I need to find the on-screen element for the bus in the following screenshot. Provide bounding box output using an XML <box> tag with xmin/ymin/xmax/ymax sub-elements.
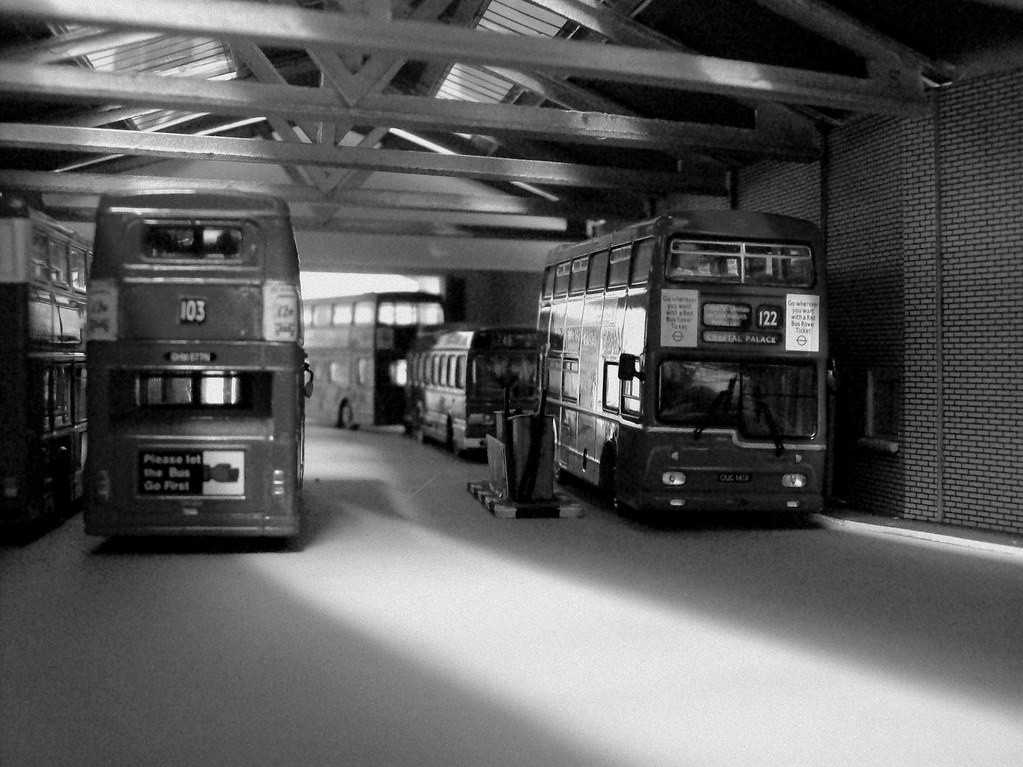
<box><xmin>297</xmin><ymin>286</ymin><xmax>448</xmax><ymax>433</ymax></box>
<box><xmin>78</xmin><ymin>185</ymin><xmax>317</xmax><ymax>542</ymax></box>
<box><xmin>402</xmin><ymin>320</ymin><xmax>539</xmax><ymax>460</ymax></box>
<box><xmin>536</xmin><ymin>207</ymin><xmax>841</xmax><ymax>517</ymax></box>
<box><xmin>0</xmin><ymin>189</ymin><xmax>94</xmax><ymax>543</ymax></box>
